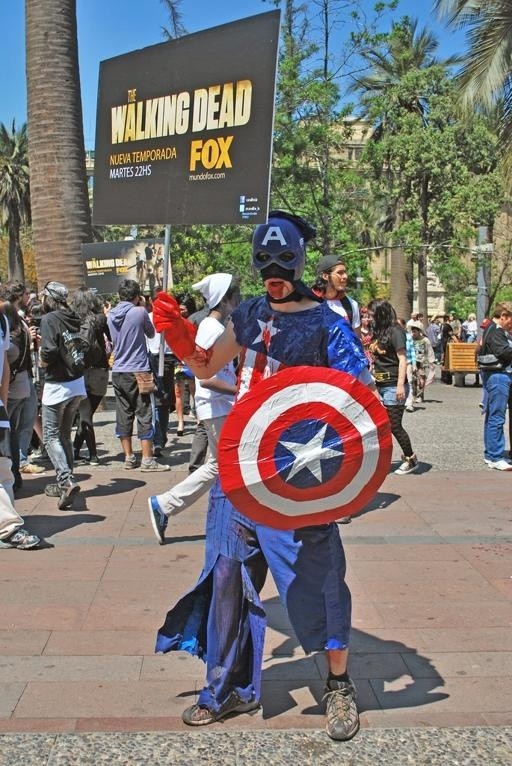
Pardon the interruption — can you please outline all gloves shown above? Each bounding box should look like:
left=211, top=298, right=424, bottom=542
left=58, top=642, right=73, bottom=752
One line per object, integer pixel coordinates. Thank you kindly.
left=150, top=288, right=202, bottom=364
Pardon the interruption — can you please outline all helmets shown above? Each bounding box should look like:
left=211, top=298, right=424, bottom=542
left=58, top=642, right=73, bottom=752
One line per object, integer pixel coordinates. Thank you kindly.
left=250, top=217, right=305, bottom=280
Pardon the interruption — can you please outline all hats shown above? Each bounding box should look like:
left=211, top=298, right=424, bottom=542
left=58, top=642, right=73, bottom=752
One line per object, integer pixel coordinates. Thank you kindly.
left=318, top=254, right=344, bottom=271
left=494, top=300, right=512, bottom=316
left=192, top=273, right=233, bottom=311
left=409, top=321, right=425, bottom=333
left=41, top=281, right=69, bottom=303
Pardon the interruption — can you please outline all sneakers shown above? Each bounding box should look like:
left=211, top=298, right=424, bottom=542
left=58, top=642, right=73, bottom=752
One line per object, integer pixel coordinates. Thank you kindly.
left=483, top=456, right=512, bottom=472
left=395, top=454, right=419, bottom=476
left=73, top=456, right=86, bottom=464
left=124, top=457, right=141, bottom=470
left=19, top=465, right=46, bottom=475
left=150, top=422, right=186, bottom=459
left=140, top=459, right=170, bottom=472
left=323, top=677, right=359, bottom=741
left=1, top=529, right=44, bottom=551
left=181, top=687, right=260, bottom=725
left=85, top=457, right=101, bottom=467
left=147, top=497, right=168, bottom=545
left=403, top=396, right=425, bottom=413
left=59, top=480, right=80, bottom=511
left=44, top=483, right=61, bottom=497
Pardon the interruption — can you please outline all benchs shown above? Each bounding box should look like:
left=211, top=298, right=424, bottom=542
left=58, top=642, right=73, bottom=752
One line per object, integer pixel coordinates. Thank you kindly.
left=449, top=342, right=485, bottom=386
left=440, top=342, right=451, bottom=382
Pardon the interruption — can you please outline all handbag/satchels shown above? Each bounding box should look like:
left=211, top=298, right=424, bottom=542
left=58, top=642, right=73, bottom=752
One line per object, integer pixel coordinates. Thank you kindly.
left=135, top=368, right=159, bottom=397
left=60, top=330, right=96, bottom=378
left=476, top=353, right=504, bottom=371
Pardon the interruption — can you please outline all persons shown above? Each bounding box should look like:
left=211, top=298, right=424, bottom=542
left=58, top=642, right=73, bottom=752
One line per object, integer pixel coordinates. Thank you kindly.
left=153, top=212, right=377, bottom=742
left=0, top=255, right=510, bottom=551
